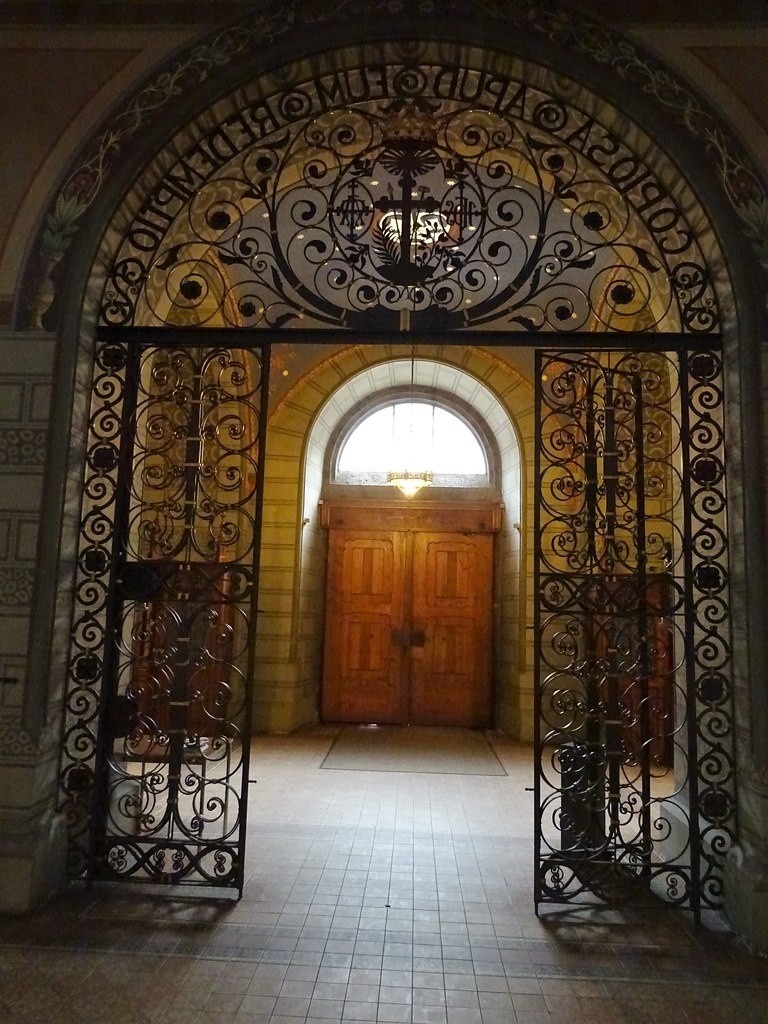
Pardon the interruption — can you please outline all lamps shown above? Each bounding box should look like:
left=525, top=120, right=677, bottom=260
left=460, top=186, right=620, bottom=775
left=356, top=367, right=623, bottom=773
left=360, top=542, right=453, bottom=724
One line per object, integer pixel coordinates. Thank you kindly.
left=387, top=402, right=434, bottom=501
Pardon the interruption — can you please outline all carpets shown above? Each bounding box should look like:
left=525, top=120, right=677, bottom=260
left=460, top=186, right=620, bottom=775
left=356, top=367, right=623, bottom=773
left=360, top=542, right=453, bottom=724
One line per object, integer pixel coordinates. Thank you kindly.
left=319, top=724, right=508, bottom=776
